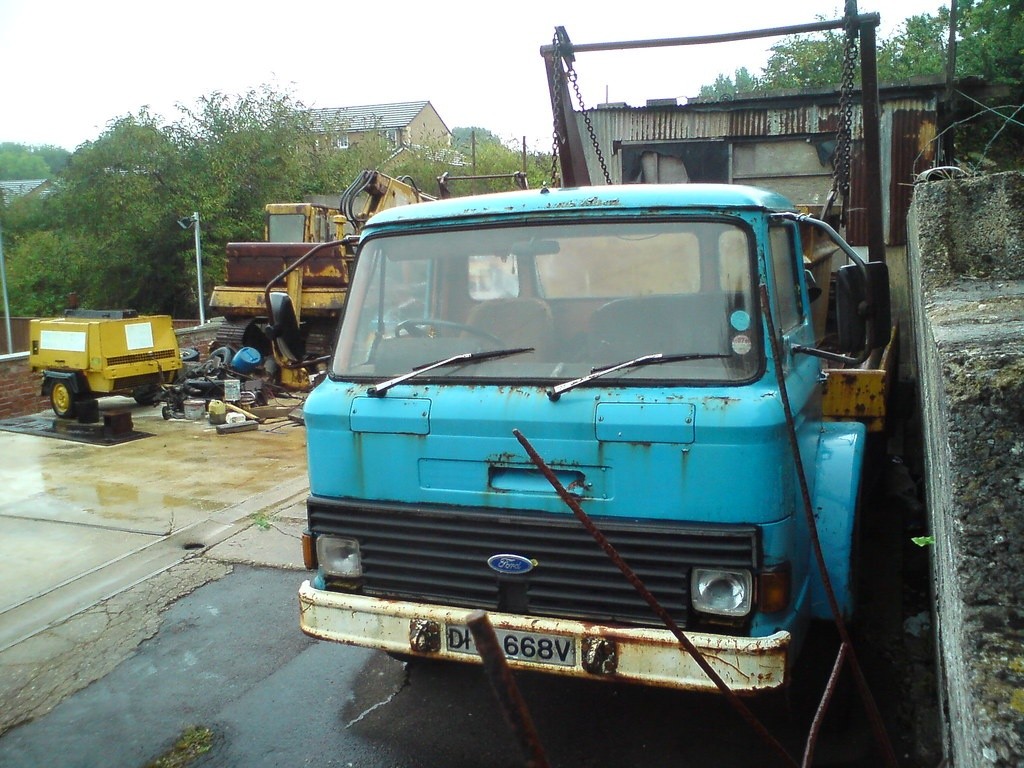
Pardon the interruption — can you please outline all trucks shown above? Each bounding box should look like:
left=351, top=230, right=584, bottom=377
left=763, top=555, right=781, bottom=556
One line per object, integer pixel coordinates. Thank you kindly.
left=292, top=181, right=902, bottom=708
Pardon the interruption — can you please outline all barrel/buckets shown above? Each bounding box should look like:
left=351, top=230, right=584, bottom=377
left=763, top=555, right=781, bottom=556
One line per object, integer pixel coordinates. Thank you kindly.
left=183, top=398, right=207, bottom=420
left=208, top=399, right=227, bottom=424
left=231, top=347, right=262, bottom=373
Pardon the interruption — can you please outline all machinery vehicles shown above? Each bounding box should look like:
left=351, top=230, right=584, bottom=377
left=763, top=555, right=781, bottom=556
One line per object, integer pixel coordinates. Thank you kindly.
left=208, top=170, right=443, bottom=391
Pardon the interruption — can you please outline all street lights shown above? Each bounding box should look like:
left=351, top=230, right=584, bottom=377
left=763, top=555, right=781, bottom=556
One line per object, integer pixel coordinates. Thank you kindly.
left=178, top=217, right=205, bottom=327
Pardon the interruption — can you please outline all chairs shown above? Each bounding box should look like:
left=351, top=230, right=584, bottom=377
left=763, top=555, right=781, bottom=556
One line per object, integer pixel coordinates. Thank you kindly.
left=593, top=296, right=672, bottom=356
left=455, top=295, right=557, bottom=375
